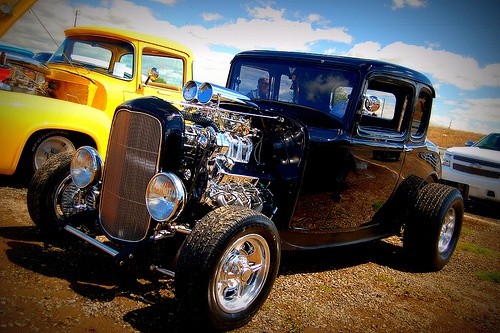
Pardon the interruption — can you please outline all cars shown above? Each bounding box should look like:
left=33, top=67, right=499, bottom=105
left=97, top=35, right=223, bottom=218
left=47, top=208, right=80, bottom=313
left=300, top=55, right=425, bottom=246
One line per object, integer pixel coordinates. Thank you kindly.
left=440, top=133, right=500, bottom=206
left=26, top=49, right=466, bottom=328
left=0, top=0, right=197, bottom=176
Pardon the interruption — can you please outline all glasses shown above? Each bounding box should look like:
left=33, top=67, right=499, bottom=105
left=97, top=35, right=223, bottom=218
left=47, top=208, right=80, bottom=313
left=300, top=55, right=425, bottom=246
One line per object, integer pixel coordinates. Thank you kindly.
left=263, top=83, right=269, bottom=87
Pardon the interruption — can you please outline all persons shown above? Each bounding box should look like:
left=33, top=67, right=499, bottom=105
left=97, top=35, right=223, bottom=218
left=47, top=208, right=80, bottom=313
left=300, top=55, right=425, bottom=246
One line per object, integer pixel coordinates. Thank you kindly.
left=246, top=76, right=269, bottom=98
